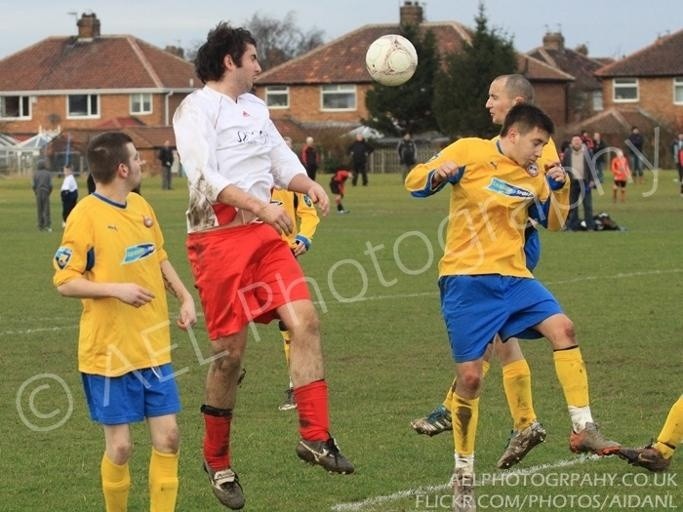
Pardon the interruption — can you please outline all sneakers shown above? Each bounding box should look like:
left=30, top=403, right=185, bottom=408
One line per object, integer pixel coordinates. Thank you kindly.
left=203, top=454, right=246, bottom=511
left=570, top=422, right=624, bottom=455
left=496, top=421, right=549, bottom=470
left=614, top=436, right=673, bottom=471
left=296, top=433, right=355, bottom=476
left=278, top=387, right=305, bottom=411
left=411, top=406, right=456, bottom=439
left=451, top=464, right=477, bottom=512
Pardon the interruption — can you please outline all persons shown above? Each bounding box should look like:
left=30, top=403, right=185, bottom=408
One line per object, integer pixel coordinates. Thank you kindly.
left=31, top=17, right=683, bottom=512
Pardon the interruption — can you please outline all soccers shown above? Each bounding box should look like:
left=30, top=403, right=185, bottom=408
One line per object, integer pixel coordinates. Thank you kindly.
left=364, top=33, right=420, bottom=87
